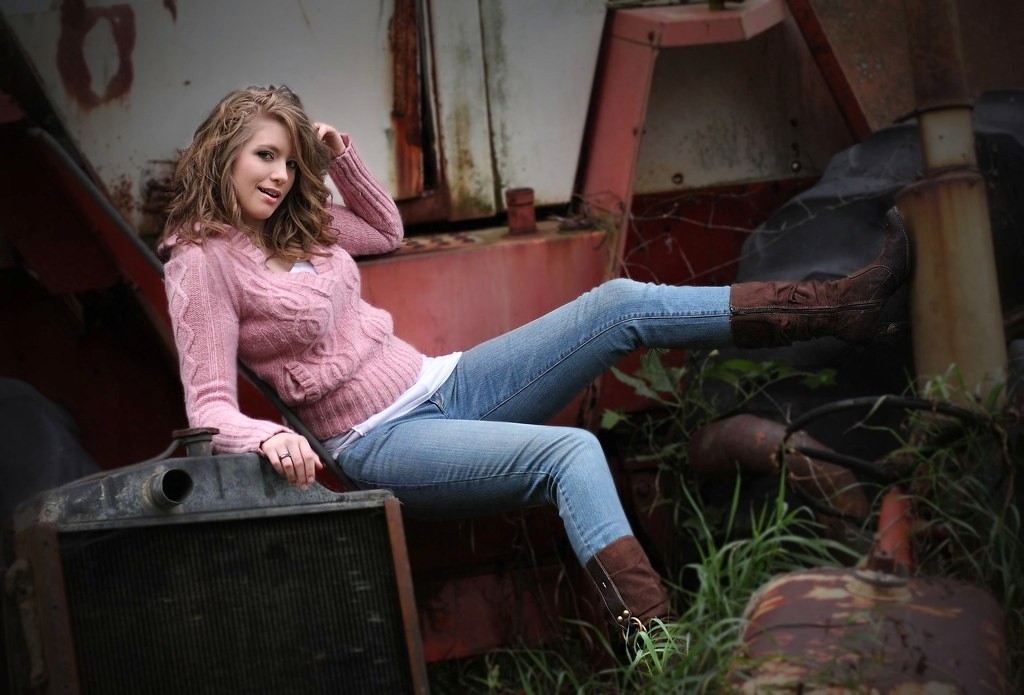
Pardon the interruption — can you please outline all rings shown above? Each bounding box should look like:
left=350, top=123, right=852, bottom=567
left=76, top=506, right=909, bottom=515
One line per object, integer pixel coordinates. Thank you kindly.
left=280, top=454, right=291, bottom=461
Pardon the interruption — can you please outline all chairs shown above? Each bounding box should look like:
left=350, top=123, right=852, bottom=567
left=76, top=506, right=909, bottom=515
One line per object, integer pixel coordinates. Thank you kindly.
left=0, top=125, right=579, bottom=576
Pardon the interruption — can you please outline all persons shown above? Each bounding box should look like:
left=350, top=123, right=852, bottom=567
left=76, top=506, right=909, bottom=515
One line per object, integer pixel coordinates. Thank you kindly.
left=158, top=83, right=910, bottom=673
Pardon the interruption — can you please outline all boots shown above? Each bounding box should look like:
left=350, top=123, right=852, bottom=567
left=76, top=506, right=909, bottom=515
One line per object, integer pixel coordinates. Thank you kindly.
left=586, top=536, right=691, bottom=686
left=728, top=210, right=913, bottom=350
